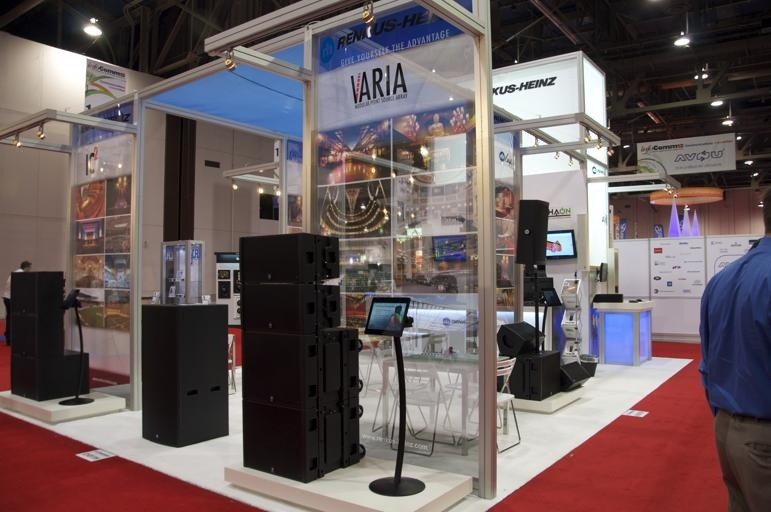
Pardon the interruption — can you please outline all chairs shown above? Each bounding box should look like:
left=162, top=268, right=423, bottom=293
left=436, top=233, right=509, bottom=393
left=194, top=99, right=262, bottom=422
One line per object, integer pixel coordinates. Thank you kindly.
left=359, top=328, right=521, bottom=456
left=228, top=334, right=237, bottom=394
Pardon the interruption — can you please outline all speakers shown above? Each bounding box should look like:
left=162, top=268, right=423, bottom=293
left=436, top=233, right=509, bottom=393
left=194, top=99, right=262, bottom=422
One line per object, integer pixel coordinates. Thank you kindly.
left=517, top=200, right=550, bottom=265
left=560, top=361, right=592, bottom=392
left=497, top=351, right=560, bottom=401
left=497, top=321, right=546, bottom=359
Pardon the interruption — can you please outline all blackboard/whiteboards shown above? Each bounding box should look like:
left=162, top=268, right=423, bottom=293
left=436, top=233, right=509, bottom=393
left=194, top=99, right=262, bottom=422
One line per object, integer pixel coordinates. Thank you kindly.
left=614, top=234, right=766, bottom=301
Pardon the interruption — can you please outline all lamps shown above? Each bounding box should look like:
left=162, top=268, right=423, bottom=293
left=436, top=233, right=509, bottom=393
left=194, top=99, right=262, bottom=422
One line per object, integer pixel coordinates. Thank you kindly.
left=37, top=125, right=46, bottom=140
left=12, top=131, right=24, bottom=149
left=81, top=18, right=104, bottom=37
left=223, top=49, right=237, bottom=71
left=651, top=12, right=766, bottom=212
left=534, top=137, right=575, bottom=167
left=360, top=1, right=376, bottom=26
left=583, top=130, right=630, bottom=156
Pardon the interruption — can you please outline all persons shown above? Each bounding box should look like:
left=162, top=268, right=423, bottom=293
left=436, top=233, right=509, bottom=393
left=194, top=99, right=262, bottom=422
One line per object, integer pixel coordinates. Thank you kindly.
left=496, top=264, right=514, bottom=287
left=389, top=305, right=401, bottom=328
left=2, top=261, right=32, bottom=346
left=429, top=261, right=457, bottom=293
left=698, top=189, right=771, bottom=512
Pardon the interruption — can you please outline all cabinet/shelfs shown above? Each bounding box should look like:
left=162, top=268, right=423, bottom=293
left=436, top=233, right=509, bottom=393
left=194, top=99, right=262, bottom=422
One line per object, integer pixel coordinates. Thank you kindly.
left=311, top=0, right=496, bottom=295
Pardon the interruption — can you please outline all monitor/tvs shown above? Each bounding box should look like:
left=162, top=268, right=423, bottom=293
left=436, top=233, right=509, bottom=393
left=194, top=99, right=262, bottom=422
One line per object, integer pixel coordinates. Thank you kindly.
left=62, top=290, right=80, bottom=310
left=364, top=297, right=410, bottom=337
left=600, top=263, right=608, bottom=282
left=524, top=277, right=553, bottom=301
left=542, top=287, right=562, bottom=306
left=545, top=230, right=577, bottom=259
left=431, top=235, right=467, bottom=262
